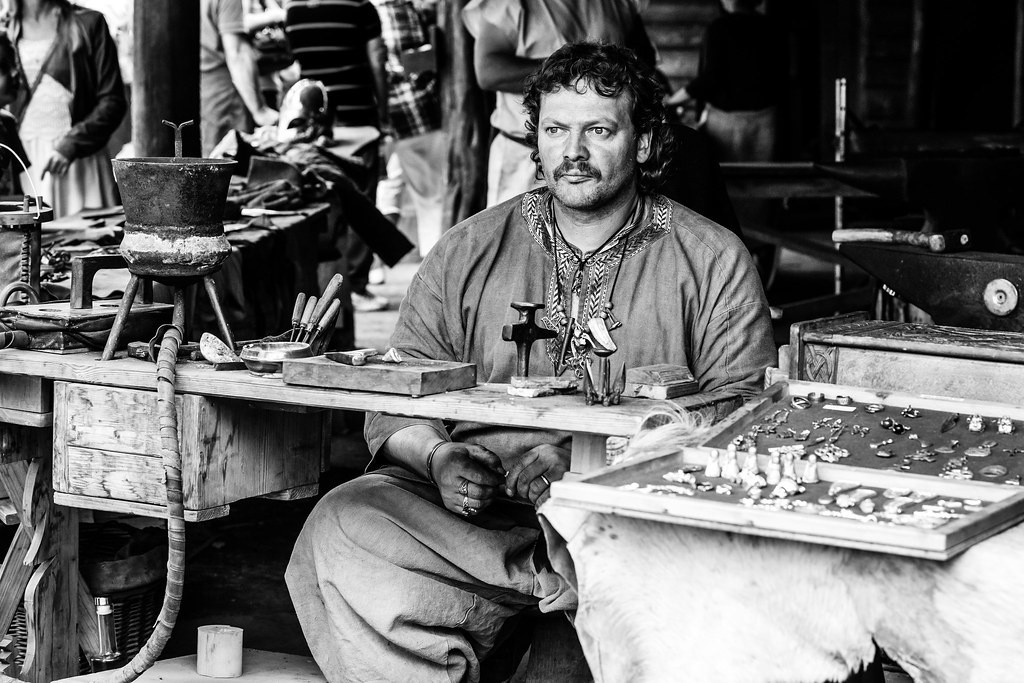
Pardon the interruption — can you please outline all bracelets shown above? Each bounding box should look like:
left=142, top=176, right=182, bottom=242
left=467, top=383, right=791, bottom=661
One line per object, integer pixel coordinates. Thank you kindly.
left=427, top=439, right=449, bottom=488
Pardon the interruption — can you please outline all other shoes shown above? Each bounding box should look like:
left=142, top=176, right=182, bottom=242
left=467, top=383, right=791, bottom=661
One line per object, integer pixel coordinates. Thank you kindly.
left=368, top=266, right=386, bottom=285
left=350, top=289, right=388, bottom=312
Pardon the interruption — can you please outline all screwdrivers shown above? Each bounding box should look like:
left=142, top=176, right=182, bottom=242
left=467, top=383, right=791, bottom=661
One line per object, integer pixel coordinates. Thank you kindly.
left=290, top=273, right=344, bottom=344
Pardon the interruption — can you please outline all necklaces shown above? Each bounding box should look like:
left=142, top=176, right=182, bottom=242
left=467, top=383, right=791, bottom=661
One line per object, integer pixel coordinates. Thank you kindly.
left=542, top=183, right=644, bottom=379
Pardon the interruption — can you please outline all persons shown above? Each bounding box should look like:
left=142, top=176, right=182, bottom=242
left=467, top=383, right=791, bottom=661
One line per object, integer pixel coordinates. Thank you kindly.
left=461, top=0, right=660, bottom=211
left=659, top=1, right=786, bottom=296
left=0, top=0, right=129, bottom=230
left=282, top=41, right=779, bottom=683
left=199, top=0, right=461, bottom=312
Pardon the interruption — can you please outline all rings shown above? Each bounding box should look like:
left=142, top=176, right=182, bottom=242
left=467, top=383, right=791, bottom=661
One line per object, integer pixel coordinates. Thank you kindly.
left=790, top=395, right=812, bottom=410
left=458, top=479, right=470, bottom=496
left=808, top=392, right=825, bottom=403
left=462, top=495, right=469, bottom=510
left=836, top=395, right=852, bottom=406
left=864, top=403, right=885, bottom=414
left=461, top=507, right=477, bottom=519
left=539, top=474, right=551, bottom=486
